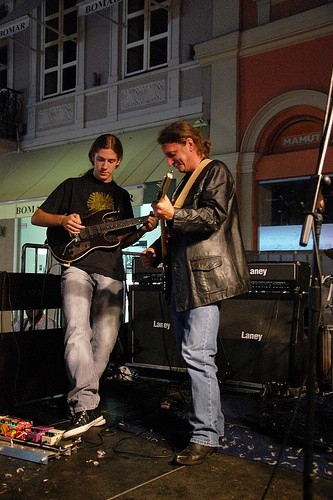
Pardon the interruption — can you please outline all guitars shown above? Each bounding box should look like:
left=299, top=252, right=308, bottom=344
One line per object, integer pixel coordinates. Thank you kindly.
left=46, top=208, right=161, bottom=264
left=153, top=170, right=174, bottom=256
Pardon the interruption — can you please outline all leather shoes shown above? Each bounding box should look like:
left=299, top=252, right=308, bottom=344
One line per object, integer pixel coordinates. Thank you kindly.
left=174, top=442, right=218, bottom=465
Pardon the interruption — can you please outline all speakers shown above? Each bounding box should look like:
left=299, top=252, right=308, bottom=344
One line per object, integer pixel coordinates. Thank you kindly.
left=128, top=280, right=318, bottom=398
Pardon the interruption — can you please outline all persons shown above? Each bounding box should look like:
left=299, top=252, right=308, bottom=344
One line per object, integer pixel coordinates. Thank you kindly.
left=31, top=134, right=159, bottom=438
left=140, top=122, right=254, bottom=463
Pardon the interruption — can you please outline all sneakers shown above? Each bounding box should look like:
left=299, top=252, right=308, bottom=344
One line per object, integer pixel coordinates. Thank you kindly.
left=63, top=408, right=106, bottom=441
